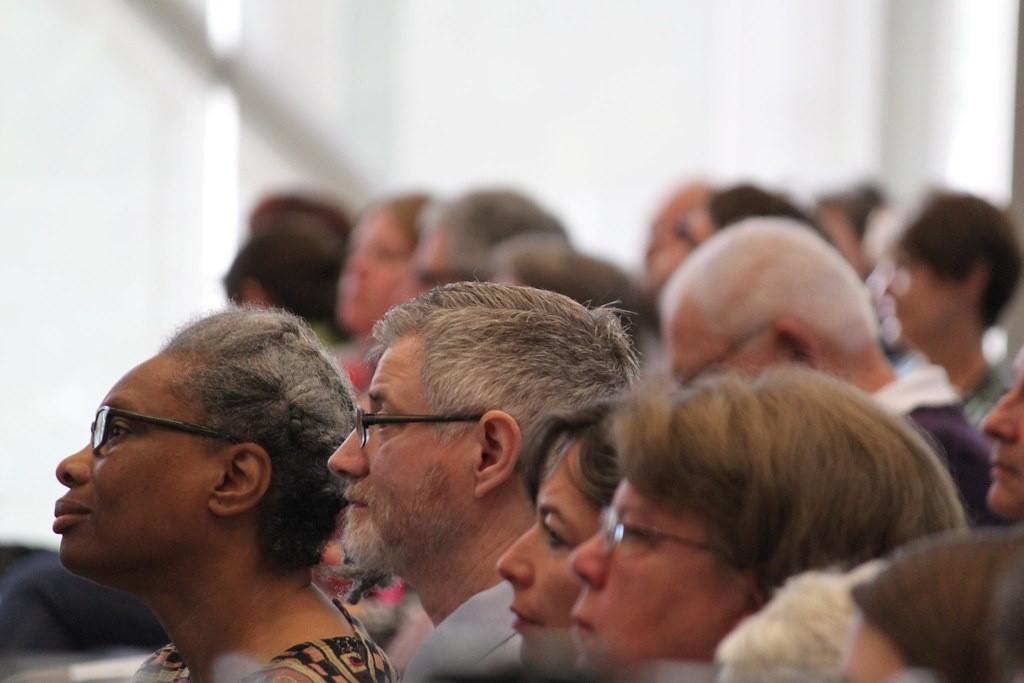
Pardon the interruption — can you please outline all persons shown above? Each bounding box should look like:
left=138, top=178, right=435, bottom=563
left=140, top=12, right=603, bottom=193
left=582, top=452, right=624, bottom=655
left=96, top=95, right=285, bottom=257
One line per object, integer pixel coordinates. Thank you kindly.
left=1, top=176, right=1024, bottom=683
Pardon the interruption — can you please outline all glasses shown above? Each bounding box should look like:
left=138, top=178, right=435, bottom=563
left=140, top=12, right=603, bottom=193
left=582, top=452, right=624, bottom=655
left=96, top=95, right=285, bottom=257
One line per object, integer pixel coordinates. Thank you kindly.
left=595, top=504, right=746, bottom=571
left=351, top=404, right=485, bottom=449
left=666, top=325, right=772, bottom=390
left=89, top=405, right=241, bottom=455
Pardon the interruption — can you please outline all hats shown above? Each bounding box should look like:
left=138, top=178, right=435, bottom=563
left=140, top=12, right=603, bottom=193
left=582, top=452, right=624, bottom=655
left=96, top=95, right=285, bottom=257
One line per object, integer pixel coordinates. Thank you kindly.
left=223, top=194, right=354, bottom=320
